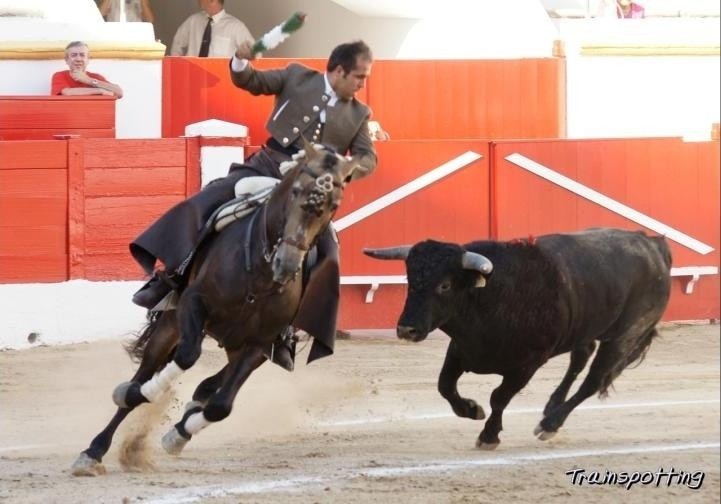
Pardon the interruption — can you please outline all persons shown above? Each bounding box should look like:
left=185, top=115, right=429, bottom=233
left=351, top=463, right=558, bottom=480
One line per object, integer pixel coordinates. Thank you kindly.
left=99, top=0, right=154, bottom=21
left=170, top=0, right=264, bottom=59
left=52, top=40, right=123, bottom=99
left=131, top=37, right=379, bottom=373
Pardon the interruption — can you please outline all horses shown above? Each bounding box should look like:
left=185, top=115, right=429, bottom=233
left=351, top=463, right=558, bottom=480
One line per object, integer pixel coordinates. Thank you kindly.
left=69, top=131, right=368, bottom=480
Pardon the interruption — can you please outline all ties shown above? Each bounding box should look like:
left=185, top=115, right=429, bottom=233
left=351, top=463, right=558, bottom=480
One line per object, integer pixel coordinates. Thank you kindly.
left=199, top=17, right=213, bottom=57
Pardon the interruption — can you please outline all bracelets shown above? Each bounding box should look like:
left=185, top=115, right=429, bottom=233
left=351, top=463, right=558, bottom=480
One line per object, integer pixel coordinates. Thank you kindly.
left=91, top=78, right=101, bottom=87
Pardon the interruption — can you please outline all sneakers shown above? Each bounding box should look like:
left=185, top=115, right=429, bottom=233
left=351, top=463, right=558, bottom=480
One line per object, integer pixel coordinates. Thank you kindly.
left=264, top=335, right=294, bottom=371
left=132, top=277, right=170, bottom=308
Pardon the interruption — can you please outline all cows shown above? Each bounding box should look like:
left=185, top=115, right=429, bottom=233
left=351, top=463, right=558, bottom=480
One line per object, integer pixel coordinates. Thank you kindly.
left=361, top=224, right=675, bottom=452
